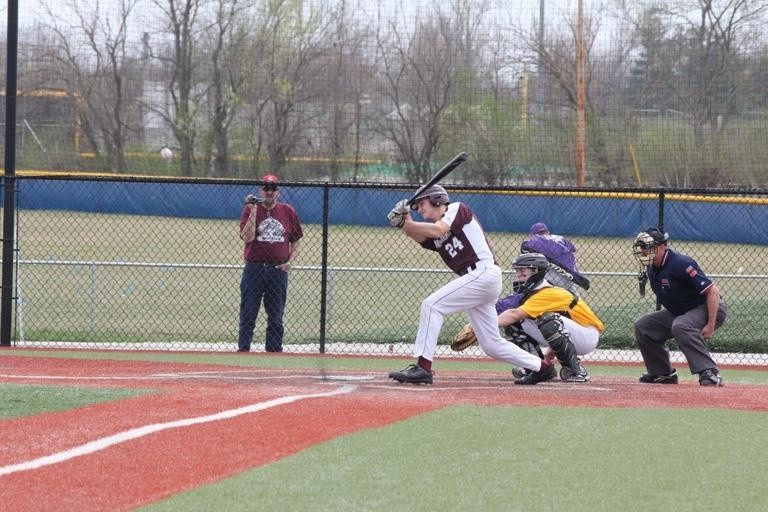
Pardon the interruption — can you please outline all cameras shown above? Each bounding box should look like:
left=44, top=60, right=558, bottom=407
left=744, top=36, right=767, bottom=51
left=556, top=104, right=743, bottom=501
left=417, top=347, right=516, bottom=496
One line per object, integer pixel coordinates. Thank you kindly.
left=249, top=196, right=264, bottom=203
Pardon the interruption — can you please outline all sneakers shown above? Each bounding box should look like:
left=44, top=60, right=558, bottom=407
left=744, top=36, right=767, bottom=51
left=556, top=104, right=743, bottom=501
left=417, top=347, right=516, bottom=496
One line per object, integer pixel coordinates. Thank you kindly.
left=512, top=363, right=589, bottom=384
left=639, top=368, right=678, bottom=384
left=389, top=364, right=433, bottom=384
left=699, top=369, right=722, bottom=385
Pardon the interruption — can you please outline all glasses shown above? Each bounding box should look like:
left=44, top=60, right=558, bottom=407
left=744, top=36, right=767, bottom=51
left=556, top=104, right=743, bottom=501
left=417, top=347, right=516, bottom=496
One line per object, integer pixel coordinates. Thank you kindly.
left=263, top=185, right=277, bottom=191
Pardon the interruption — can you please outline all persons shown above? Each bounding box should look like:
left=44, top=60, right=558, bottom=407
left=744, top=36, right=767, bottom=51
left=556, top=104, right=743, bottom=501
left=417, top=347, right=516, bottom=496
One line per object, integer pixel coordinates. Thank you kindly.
left=512, top=223, right=578, bottom=378
left=387, top=184, right=557, bottom=384
left=237, top=175, right=303, bottom=353
left=632, top=228, right=728, bottom=387
left=450, top=253, right=604, bottom=382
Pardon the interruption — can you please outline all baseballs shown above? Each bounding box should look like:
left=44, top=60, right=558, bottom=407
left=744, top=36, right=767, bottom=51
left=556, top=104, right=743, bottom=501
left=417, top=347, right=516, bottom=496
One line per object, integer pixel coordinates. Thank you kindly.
left=161, top=147, right=172, bottom=159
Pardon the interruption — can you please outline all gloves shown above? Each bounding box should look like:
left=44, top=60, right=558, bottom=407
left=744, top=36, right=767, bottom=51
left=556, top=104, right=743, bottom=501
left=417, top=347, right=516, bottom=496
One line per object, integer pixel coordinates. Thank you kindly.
left=395, top=199, right=410, bottom=214
left=387, top=209, right=403, bottom=226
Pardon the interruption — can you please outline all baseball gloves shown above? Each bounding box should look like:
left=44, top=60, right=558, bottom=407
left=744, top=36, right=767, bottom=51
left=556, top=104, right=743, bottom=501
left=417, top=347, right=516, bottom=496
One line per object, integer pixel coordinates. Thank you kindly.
left=451, top=323, right=476, bottom=351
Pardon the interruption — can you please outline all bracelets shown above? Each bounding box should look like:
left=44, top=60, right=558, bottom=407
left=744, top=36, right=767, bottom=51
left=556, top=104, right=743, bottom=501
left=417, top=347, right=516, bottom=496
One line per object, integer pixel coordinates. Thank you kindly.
left=287, top=261, right=291, bottom=266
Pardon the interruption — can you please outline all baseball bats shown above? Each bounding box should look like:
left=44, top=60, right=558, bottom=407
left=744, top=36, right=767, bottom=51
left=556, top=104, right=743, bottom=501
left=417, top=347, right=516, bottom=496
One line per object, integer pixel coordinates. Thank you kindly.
left=407, top=151, right=470, bottom=207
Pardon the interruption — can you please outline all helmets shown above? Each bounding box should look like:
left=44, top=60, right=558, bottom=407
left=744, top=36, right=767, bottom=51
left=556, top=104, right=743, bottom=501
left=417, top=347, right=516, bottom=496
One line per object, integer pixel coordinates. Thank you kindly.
left=415, top=185, right=449, bottom=206
left=631, top=227, right=667, bottom=273
left=512, top=252, right=548, bottom=294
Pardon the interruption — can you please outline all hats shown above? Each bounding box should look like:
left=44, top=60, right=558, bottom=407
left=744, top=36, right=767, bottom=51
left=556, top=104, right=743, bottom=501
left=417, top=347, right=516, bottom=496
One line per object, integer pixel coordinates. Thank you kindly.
left=263, top=175, right=278, bottom=182
left=530, top=223, right=547, bottom=234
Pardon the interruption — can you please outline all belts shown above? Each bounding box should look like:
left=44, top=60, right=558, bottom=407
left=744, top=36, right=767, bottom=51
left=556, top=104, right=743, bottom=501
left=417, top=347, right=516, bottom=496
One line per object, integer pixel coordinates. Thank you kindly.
left=251, top=261, right=284, bottom=267
left=459, top=263, right=476, bottom=276
